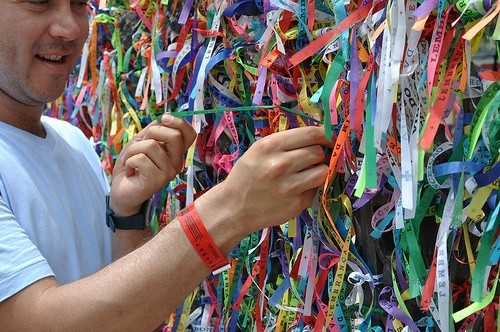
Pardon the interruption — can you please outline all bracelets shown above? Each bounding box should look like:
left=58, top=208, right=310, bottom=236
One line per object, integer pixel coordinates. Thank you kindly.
left=176, top=202, right=232, bottom=276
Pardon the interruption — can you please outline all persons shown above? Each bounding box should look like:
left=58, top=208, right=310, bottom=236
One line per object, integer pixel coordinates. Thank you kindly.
left=1, top=1, right=332, bottom=331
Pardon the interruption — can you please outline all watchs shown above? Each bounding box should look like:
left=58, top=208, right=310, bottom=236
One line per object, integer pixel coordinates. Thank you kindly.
left=105, top=189, right=157, bottom=232
